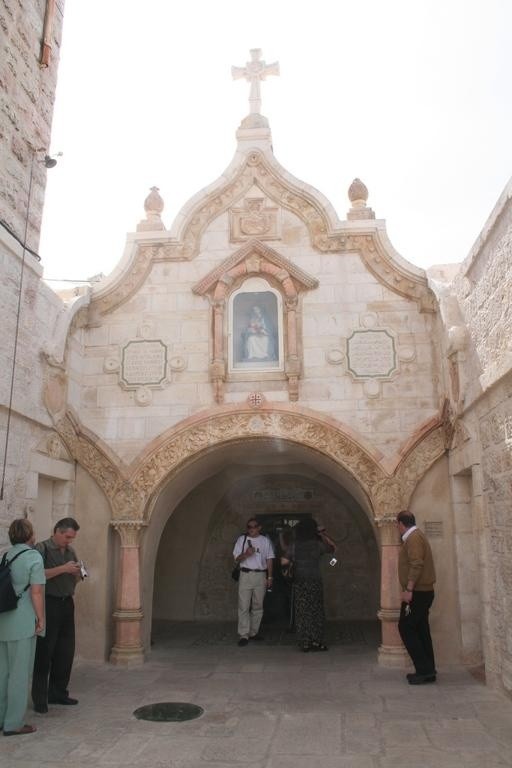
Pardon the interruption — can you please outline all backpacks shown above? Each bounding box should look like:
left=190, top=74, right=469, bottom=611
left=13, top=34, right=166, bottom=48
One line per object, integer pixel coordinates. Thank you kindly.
left=0, top=548, right=36, bottom=613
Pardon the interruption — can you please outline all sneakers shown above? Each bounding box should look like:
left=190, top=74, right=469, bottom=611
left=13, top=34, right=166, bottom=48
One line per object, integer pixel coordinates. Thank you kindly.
left=35, top=702, right=49, bottom=713
left=247, top=634, right=265, bottom=641
left=238, top=638, right=248, bottom=646
left=407, top=672, right=436, bottom=684
left=48, top=697, right=78, bottom=705
left=0, top=724, right=37, bottom=735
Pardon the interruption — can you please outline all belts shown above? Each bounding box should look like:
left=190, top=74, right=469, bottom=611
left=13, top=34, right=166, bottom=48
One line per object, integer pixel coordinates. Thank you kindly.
left=241, top=567, right=267, bottom=572
left=45, top=594, right=73, bottom=601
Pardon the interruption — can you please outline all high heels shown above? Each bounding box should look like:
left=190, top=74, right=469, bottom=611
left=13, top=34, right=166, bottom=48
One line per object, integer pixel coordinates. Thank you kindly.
left=303, top=640, right=328, bottom=652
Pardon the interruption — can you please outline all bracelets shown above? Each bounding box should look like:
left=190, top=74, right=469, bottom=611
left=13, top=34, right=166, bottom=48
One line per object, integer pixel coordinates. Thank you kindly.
left=406, top=588, right=413, bottom=592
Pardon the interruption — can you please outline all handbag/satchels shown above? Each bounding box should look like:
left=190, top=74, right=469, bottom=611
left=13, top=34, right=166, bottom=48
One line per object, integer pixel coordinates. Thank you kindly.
left=278, top=560, right=294, bottom=581
left=232, top=565, right=239, bottom=580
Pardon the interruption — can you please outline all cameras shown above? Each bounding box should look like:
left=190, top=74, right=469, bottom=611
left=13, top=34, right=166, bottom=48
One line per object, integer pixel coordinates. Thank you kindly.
left=74, top=560, right=88, bottom=582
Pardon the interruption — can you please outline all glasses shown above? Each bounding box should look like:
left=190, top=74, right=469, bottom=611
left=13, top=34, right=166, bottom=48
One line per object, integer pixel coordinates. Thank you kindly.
left=247, top=524, right=259, bottom=529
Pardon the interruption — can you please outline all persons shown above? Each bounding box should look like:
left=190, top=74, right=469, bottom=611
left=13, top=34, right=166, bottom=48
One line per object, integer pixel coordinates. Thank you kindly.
left=30, top=518, right=84, bottom=713
left=231, top=518, right=337, bottom=653
left=0, top=519, right=47, bottom=736
left=396, top=510, right=437, bottom=685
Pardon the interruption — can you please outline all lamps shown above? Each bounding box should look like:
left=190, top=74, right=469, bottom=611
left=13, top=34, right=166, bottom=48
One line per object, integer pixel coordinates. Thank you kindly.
left=36, top=147, right=57, bottom=168
